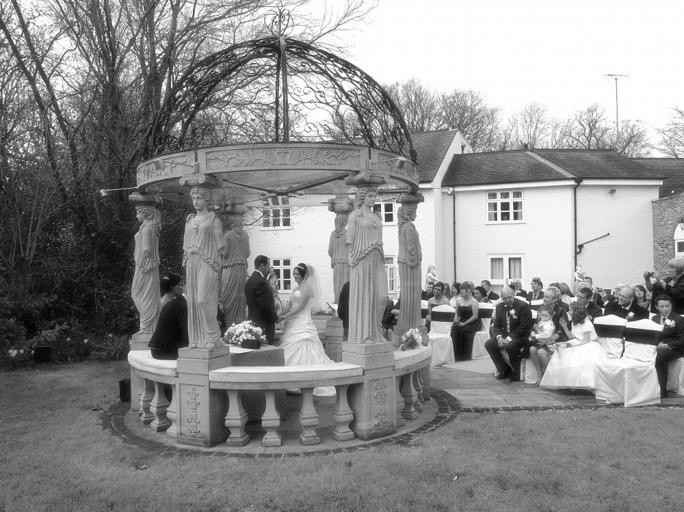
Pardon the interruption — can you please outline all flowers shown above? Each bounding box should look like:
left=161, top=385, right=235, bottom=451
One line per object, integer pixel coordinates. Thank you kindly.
left=223, top=318, right=268, bottom=346
left=398, top=326, right=423, bottom=350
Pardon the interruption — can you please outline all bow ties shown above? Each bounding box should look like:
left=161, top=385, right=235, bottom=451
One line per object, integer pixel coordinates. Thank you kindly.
left=505, top=306, right=513, bottom=311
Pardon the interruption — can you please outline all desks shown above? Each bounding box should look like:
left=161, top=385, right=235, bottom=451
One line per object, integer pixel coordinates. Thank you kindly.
left=220, top=336, right=284, bottom=366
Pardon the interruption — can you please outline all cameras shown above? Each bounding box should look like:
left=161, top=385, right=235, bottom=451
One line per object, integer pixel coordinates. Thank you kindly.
left=647, top=271, right=655, bottom=277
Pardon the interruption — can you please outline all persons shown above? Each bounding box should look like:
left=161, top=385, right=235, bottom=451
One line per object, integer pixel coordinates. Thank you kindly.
left=426, top=267, right=439, bottom=284
left=148, top=273, right=187, bottom=360
left=182, top=187, right=252, bottom=349
left=244, top=255, right=278, bottom=346
left=574, top=265, right=589, bottom=284
left=130, top=205, right=161, bottom=336
left=642, top=257, right=683, bottom=314
left=327, top=186, right=390, bottom=343
left=397, top=203, right=422, bottom=331
left=278, top=263, right=339, bottom=396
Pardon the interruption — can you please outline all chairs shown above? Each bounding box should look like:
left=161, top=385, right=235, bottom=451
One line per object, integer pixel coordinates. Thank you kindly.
left=420, top=295, right=684, bottom=411
